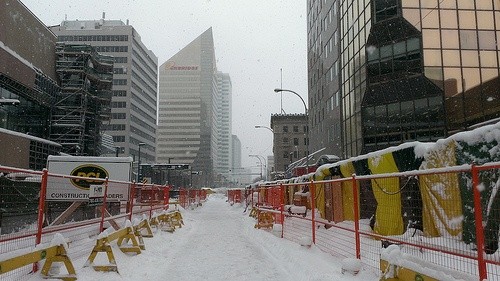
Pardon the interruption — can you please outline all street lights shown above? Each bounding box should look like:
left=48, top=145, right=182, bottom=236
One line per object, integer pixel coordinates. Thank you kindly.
left=274, top=89, right=308, bottom=173
left=250, top=154, right=263, bottom=179
left=253, top=155, right=267, bottom=182
left=254, top=125, right=277, bottom=180
left=168, top=157, right=173, bottom=165
left=138, top=142, right=146, bottom=184
